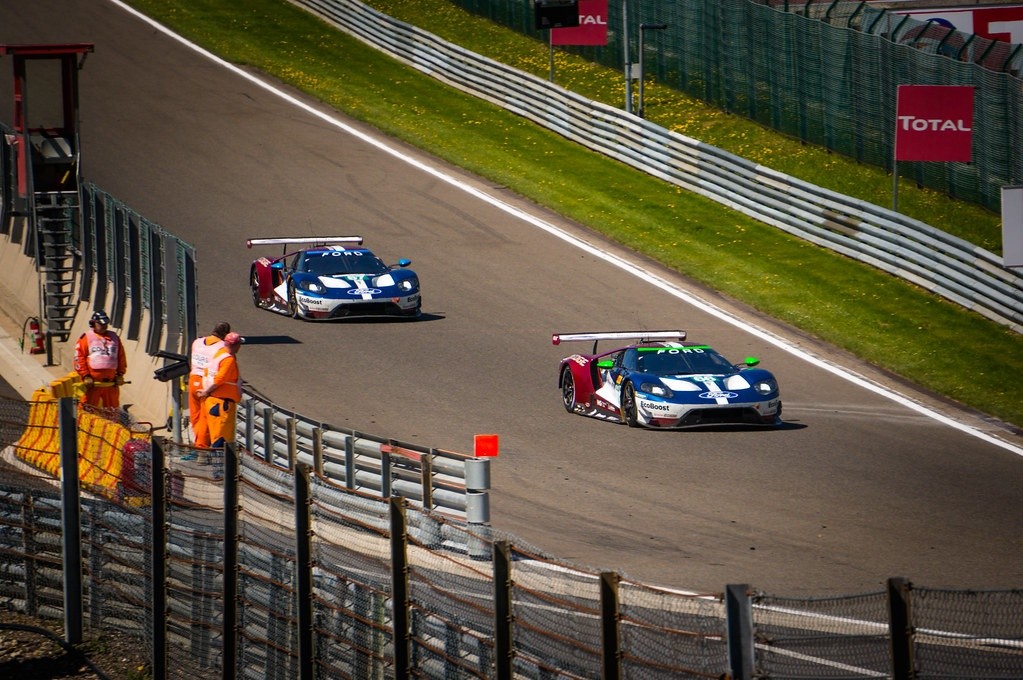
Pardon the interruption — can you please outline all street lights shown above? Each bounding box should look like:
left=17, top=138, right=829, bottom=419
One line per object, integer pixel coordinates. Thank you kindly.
left=636, top=23, right=669, bottom=118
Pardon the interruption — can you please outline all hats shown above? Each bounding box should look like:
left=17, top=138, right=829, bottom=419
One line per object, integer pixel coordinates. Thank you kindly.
left=92, top=311, right=109, bottom=325
left=224, top=332, right=245, bottom=345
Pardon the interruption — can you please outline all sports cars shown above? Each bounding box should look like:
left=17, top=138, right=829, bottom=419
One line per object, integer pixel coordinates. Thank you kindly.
left=551, top=329, right=785, bottom=432
left=245, top=234, right=424, bottom=324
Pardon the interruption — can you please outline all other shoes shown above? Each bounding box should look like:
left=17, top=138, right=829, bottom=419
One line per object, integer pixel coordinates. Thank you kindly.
left=197, top=452, right=212, bottom=467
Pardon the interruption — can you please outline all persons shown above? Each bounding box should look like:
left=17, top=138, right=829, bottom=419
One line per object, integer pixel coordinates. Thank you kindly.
left=188, top=321, right=231, bottom=466
left=74, top=311, right=128, bottom=421
left=196, top=333, right=245, bottom=482
